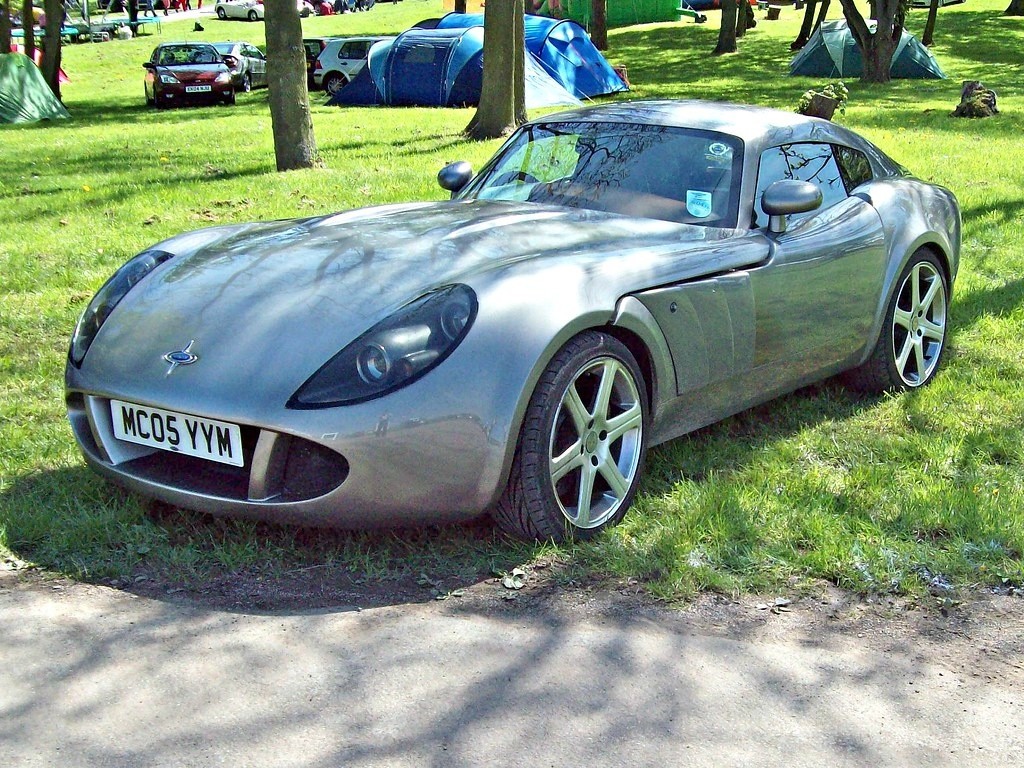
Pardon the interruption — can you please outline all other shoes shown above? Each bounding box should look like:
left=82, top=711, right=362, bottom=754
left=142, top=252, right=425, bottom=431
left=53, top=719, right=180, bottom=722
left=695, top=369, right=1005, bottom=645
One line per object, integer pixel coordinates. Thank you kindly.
left=166, top=14, right=168, bottom=16
left=144, top=14, right=148, bottom=17
left=163, top=11, right=165, bottom=15
left=154, top=15, right=157, bottom=17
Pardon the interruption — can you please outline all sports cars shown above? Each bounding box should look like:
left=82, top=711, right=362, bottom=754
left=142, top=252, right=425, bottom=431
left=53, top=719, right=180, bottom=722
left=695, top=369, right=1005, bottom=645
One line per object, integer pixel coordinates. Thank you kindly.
left=63, top=96, right=964, bottom=547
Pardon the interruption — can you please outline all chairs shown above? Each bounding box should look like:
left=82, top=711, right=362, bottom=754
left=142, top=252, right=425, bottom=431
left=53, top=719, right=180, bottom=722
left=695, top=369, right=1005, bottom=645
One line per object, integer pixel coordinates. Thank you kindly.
left=10, top=15, right=161, bottom=48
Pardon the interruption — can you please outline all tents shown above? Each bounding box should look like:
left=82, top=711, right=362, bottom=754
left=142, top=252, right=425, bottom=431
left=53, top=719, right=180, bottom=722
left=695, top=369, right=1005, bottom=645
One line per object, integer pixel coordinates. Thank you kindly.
left=786, top=19, right=947, bottom=80
left=322, top=26, right=596, bottom=110
left=10, top=44, right=71, bottom=84
left=411, top=12, right=632, bottom=100
left=0, top=51, right=73, bottom=124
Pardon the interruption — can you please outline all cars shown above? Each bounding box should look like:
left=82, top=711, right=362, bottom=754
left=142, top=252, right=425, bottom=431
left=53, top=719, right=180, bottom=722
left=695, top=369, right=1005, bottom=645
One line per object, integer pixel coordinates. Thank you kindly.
left=907, top=0, right=967, bottom=8
left=215, top=0, right=376, bottom=22
left=186, top=39, right=267, bottom=93
left=304, top=34, right=347, bottom=76
left=141, top=42, right=237, bottom=109
left=312, top=36, right=400, bottom=97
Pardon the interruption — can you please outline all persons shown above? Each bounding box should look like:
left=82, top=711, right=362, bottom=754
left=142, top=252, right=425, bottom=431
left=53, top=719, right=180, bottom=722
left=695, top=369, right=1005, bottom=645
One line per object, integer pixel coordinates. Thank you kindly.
left=144, top=0, right=157, bottom=17
left=162, top=0, right=170, bottom=16
left=175, top=0, right=202, bottom=13
